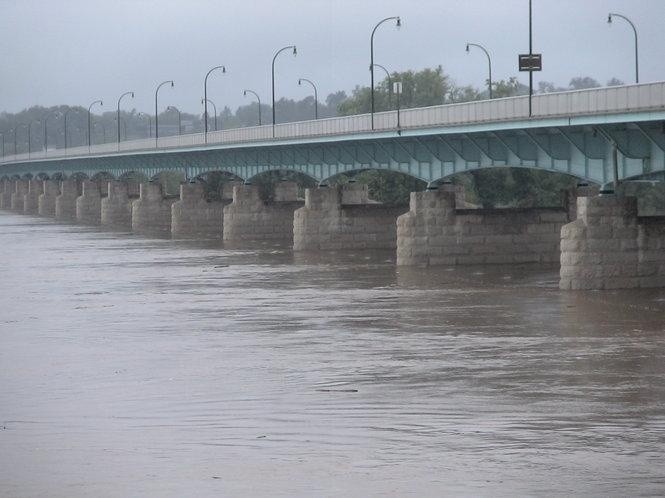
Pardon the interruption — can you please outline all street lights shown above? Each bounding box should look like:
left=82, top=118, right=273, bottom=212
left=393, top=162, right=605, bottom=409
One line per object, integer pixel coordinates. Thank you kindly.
left=272, top=45, right=297, bottom=124
left=298, top=78, right=318, bottom=119
left=243, top=89, right=261, bottom=127
left=369, top=16, right=402, bottom=112
left=466, top=42, right=494, bottom=99
left=201, top=97, right=217, bottom=130
left=204, top=65, right=225, bottom=132
left=369, top=63, right=392, bottom=110
left=607, top=13, right=640, bottom=84
left=0, top=80, right=182, bottom=158
